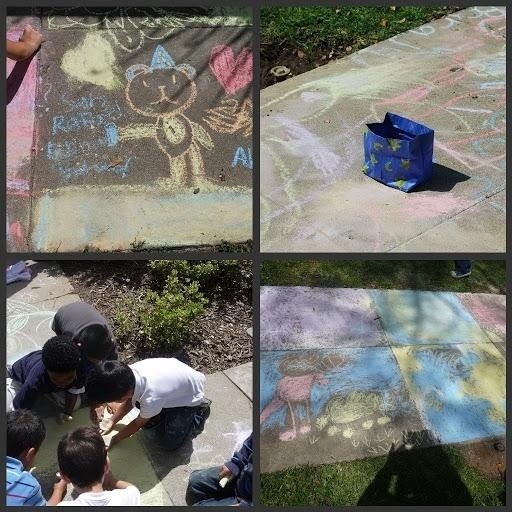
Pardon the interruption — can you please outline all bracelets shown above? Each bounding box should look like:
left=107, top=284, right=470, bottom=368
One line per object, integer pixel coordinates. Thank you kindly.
left=65, top=412, right=71, bottom=415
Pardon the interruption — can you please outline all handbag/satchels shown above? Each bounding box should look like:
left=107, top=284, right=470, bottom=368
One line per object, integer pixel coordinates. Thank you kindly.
left=361, top=111, right=435, bottom=194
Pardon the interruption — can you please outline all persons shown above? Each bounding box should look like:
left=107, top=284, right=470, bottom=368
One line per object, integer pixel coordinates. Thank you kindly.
left=7, top=24, right=43, bottom=61
left=52, top=301, right=121, bottom=423
left=6, top=334, right=87, bottom=422
left=88, top=357, right=213, bottom=451
left=6, top=411, right=67, bottom=506
left=54, top=425, right=141, bottom=506
left=188, top=432, right=254, bottom=507
